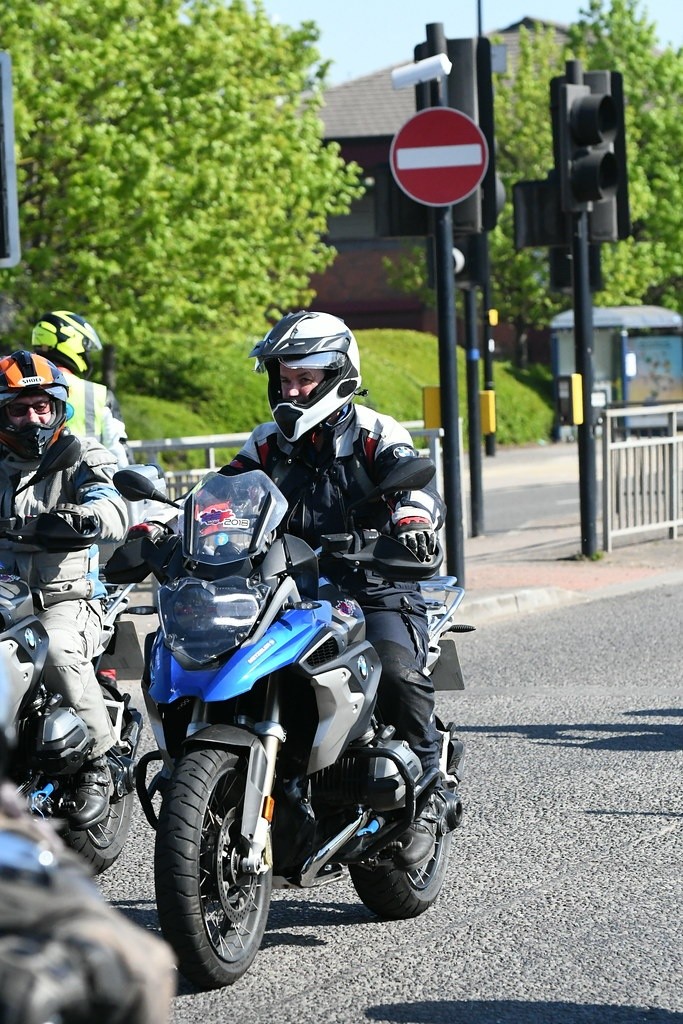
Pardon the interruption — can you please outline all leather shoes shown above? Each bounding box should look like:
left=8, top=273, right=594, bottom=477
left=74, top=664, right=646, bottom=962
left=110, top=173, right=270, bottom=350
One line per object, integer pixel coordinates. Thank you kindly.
left=64, top=758, right=117, bottom=832
left=387, top=789, right=449, bottom=870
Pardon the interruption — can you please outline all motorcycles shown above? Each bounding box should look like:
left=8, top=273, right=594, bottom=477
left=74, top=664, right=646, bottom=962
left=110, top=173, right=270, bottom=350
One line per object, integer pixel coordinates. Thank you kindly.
left=101, top=448, right=478, bottom=989
left=1, top=510, right=153, bottom=873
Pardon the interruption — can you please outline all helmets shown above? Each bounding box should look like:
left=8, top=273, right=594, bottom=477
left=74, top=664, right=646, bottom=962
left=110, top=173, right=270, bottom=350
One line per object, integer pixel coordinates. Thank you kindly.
left=247, top=310, right=362, bottom=443
left=31, top=309, right=102, bottom=381
left=0, top=351, right=68, bottom=460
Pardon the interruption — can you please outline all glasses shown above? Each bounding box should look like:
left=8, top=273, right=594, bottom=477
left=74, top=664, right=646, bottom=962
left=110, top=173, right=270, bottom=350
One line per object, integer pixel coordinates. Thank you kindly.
left=4, top=401, right=57, bottom=419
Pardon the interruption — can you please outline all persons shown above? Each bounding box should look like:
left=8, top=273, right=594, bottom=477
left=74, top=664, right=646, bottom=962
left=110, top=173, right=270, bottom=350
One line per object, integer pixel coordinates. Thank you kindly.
left=25, top=310, right=131, bottom=487
left=0, top=356, right=152, bottom=802
left=134, top=307, right=468, bottom=870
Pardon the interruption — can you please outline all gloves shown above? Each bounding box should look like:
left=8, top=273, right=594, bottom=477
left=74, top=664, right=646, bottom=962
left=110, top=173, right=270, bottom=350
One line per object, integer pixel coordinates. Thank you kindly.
left=378, top=496, right=439, bottom=564
left=119, top=516, right=176, bottom=553
left=49, top=500, right=95, bottom=537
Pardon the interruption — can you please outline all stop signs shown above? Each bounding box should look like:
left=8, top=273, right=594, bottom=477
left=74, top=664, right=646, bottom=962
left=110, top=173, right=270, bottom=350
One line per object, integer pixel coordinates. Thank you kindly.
left=389, top=106, right=492, bottom=209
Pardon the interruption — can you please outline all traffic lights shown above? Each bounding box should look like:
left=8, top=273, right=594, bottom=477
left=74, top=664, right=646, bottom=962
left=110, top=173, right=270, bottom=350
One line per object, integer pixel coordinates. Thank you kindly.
left=556, top=80, right=621, bottom=214
